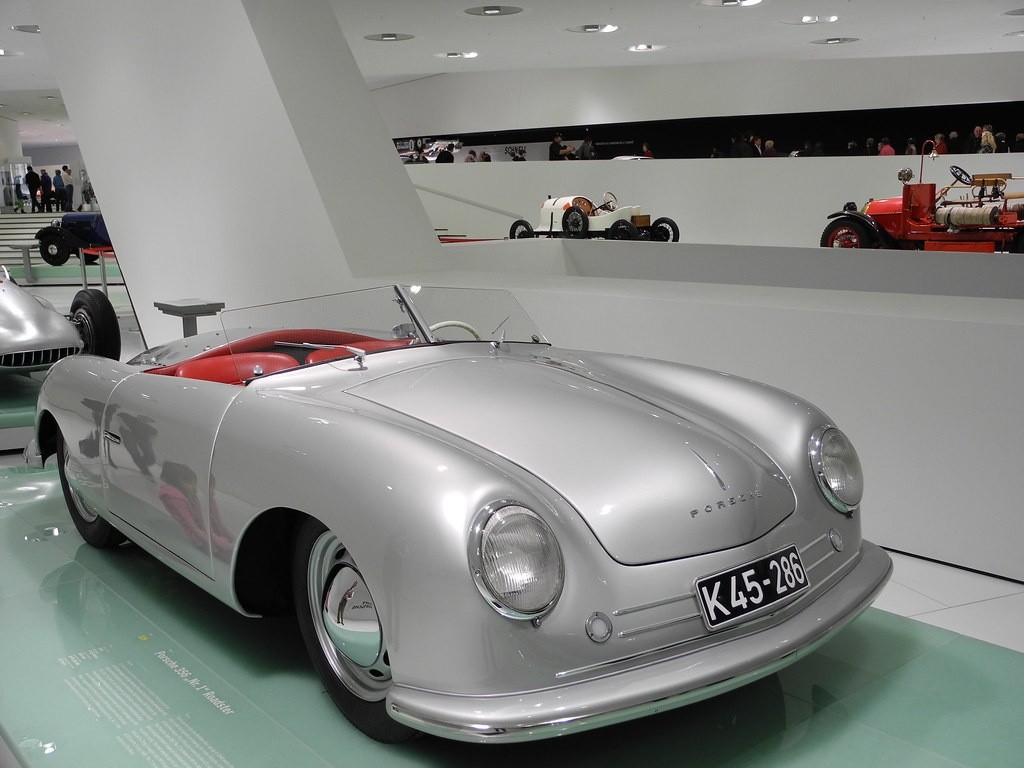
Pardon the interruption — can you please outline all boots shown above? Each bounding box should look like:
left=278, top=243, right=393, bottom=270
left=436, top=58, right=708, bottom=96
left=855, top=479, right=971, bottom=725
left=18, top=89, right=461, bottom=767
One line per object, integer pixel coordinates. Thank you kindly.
left=21, top=209, right=27, bottom=213
left=13, top=208, right=19, bottom=213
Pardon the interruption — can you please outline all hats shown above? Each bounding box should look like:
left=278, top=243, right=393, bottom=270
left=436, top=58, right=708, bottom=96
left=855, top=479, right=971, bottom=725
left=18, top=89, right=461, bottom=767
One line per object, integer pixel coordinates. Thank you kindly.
left=554, top=133, right=562, bottom=137
left=908, top=138, right=915, bottom=144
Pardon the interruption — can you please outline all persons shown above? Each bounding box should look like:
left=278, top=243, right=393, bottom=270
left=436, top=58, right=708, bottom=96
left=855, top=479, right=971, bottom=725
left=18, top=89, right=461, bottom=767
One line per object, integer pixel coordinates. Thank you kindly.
left=40, top=165, right=76, bottom=212
left=879, top=137, right=895, bottom=155
left=640, top=142, right=654, bottom=157
left=465, top=150, right=491, bottom=162
left=948, top=132, right=963, bottom=154
left=408, top=147, right=430, bottom=163
left=803, top=141, right=825, bottom=155
left=846, top=141, right=859, bottom=153
left=13, top=184, right=28, bottom=213
left=510, top=149, right=527, bottom=161
left=579, top=134, right=600, bottom=160
left=762, top=139, right=776, bottom=154
left=726, top=128, right=764, bottom=157
left=932, top=133, right=948, bottom=154
left=864, top=138, right=878, bottom=156
left=964, top=125, right=1024, bottom=154
left=25, top=167, right=41, bottom=212
left=435, top=144, right=455, bottom=163
left=548, top=132, right=576, bottom=160
left=905, top=138, right=917, bottom=155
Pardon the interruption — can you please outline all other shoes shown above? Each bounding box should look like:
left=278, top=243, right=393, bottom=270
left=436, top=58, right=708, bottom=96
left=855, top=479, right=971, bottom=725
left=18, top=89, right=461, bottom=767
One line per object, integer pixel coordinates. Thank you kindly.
left=56, top=209, right=59, bottom=212
left=31, top=211, right=35, bottom=213
left=70, top=210, right=76, bottom=212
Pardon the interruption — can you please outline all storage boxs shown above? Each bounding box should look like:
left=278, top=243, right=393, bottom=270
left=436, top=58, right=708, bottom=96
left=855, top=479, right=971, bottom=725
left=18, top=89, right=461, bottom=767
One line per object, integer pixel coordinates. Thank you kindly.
left=631, top=213, right=651, bottom=228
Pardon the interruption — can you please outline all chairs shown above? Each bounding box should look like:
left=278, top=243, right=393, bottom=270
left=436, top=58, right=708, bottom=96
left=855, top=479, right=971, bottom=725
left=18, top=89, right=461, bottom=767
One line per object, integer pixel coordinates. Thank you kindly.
left=306, top=340, right=411, bottom=364
left=176, top=352, right=299, bottom=385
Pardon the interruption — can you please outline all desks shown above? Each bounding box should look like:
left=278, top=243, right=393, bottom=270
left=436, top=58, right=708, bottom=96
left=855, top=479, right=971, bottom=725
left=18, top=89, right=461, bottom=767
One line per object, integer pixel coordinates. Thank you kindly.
left=8, top=242, right=39, bottom=282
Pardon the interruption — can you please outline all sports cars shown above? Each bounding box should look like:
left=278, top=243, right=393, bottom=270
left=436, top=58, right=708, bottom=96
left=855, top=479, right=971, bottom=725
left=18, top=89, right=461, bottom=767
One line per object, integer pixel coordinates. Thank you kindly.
left=24, top=285, right=894, bottom=745
left=0, top=263, right=121, bottom=373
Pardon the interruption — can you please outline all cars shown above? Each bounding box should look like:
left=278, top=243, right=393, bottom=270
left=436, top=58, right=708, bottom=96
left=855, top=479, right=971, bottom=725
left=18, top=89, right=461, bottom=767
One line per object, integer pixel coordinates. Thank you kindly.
left=610, top=155, right=654, bottom=160
left=789, top=150, right=807, bottom=157
left=34, top=212, right=116, bottom=266
left=821, top=139, right=1024, bottom=255
left=509, top=191, right=679, bottom=242
